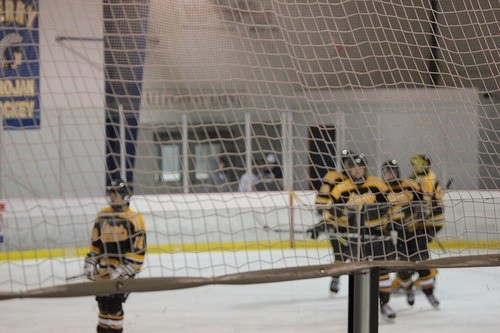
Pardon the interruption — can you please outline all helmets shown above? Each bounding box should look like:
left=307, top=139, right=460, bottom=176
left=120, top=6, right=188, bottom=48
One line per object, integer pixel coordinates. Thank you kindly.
left=107, top=170, right=134, bottom=210
left=341, top=150, right=367, bottom=185
left=412, top=152, right=431, bottom=176
left=381, top=160, right=400, bottom=183
left=411, top=155, right=432, bottom=166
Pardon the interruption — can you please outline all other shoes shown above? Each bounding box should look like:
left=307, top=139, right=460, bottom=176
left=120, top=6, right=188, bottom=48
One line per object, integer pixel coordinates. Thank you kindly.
left=408, top=293, right=414, bottom=305
left=381, top=304, right=397, bottom=323
left=330, top=278, right=339, bottom=293
left=427, top=294, right=440, bottom=311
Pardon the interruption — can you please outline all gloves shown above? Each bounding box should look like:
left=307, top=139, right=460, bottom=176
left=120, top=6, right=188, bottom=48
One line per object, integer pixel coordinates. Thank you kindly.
left=110, top=263, right=136, bottom=279
left=83, top=257, right=100, bottom=281
left=306, top=222, right=326, bottom=238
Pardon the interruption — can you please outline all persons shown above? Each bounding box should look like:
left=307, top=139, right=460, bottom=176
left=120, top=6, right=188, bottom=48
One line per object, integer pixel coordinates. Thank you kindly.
left=82, top=177, right=147, bottom=333
left=238, top=159, right=259, bottom=192
left=315, top=150, right=445, bottom=318
left=262, top=153, right=284, bottom=191
